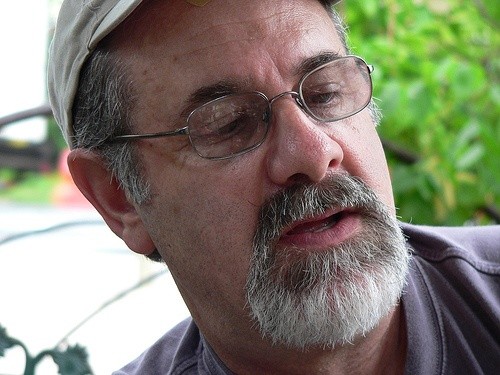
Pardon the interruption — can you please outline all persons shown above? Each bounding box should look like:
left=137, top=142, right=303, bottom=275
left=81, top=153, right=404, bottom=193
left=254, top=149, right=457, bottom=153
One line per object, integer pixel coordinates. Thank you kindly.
left=45, top=1, right=500, bottom=375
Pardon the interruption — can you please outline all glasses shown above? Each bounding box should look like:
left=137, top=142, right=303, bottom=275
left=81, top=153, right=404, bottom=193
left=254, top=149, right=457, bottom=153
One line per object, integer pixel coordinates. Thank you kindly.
left=102, top=52, right=376, bottom=161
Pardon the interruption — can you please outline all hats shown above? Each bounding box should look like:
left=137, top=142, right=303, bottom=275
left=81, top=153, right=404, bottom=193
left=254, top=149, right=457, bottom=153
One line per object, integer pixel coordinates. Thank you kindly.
left=44, top=1, right=345, bottom=151
